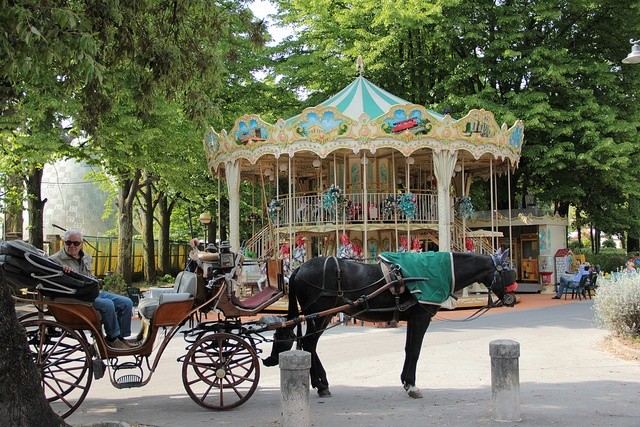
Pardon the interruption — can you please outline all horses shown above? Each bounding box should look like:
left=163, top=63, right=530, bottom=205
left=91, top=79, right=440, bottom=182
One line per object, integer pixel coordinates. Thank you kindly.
left=261, top=246, right=518, bottom=399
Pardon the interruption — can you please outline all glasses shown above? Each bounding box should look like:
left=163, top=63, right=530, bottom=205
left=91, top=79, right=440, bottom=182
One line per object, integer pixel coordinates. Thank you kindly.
left=63, top=240, right=81, bottom=246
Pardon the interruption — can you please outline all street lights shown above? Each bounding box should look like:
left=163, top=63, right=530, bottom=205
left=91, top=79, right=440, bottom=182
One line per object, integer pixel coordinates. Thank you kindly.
left=199, top=212, right=212, bottom=251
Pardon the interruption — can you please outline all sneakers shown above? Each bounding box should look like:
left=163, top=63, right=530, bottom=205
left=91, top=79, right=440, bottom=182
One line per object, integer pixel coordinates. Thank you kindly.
left=105, top=338, right=129, bottom=349
left=121, top=337, right=139, bottom=347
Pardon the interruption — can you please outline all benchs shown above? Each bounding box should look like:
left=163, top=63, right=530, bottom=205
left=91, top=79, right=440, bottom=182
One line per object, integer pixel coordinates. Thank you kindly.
left=48, top=259, right=284, bottom=329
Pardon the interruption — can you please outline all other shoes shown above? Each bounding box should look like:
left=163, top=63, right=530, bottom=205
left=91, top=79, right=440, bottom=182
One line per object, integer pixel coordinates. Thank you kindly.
left=552, top=296, right=560, bottom=299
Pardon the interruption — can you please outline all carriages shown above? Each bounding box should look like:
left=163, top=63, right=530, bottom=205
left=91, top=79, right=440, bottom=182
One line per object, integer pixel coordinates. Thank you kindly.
left=0, top=230, right=520, bottom=418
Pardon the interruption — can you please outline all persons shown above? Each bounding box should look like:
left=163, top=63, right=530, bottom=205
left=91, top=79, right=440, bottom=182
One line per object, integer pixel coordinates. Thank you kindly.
left=45, top=229, right=139, bottom=350
left=551, top=264, right=594, bottom=299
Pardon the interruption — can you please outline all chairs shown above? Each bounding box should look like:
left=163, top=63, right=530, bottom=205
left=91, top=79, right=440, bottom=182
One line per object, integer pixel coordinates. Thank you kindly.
left=564, top=273, right=600, bottom=300
